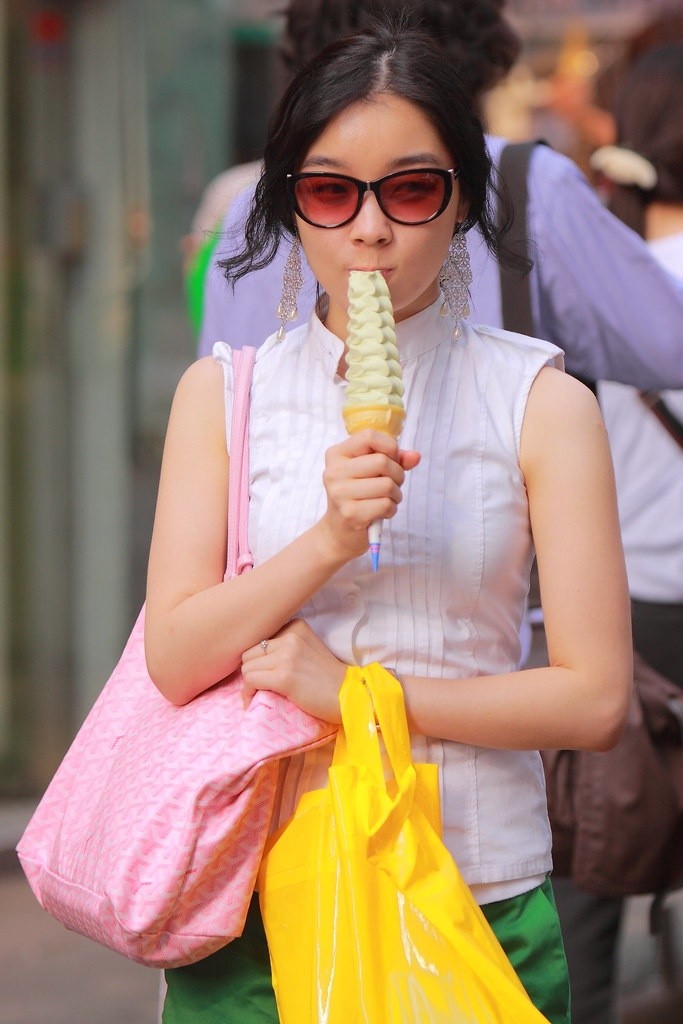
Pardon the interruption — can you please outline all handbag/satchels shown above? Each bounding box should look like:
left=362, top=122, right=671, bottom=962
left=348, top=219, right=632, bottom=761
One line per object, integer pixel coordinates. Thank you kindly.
left=539, top=653, right=683, bottom=895
left=16, top=347, right=348, bottom=967
left=260, top=665, right=558, bottom=1024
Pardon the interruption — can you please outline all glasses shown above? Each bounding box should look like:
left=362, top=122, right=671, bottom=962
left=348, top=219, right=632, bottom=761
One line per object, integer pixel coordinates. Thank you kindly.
left=285, top=168, right=458, bottom=229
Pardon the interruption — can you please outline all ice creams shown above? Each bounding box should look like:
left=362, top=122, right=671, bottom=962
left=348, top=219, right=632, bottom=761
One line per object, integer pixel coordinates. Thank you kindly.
left=343, top=271, right=406, bottom=437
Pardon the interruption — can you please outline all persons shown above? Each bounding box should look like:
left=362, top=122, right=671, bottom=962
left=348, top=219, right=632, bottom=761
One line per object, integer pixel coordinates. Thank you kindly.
left=143, top=25, right=632, bottom=1024
left=177, top=1, right=682, bottom=1024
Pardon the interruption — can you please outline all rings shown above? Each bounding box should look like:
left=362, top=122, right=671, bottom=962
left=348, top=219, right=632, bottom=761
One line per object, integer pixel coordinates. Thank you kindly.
left=262, top=640, right=269, bottom=655
